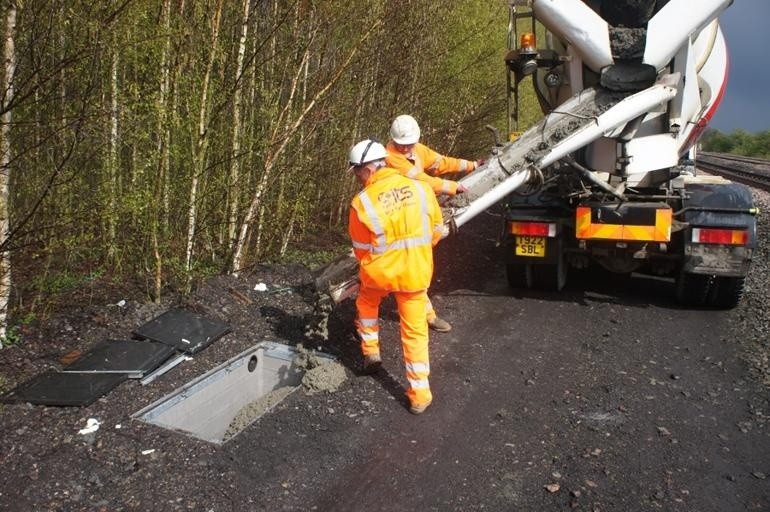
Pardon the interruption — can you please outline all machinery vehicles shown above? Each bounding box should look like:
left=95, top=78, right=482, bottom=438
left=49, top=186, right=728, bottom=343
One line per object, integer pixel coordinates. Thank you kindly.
left=317, top=1, right=759, bottom=317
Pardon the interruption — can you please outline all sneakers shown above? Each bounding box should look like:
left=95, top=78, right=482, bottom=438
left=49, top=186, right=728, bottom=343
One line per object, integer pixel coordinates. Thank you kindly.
left=428, top=317, right=452, bottom=332
left=410, top=399, right=431, bottom=414
left=364, top=352, right=382, bottom=374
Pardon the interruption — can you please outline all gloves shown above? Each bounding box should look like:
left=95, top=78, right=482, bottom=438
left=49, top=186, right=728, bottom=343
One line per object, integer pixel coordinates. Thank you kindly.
left=456, top=184, right=468, bottom=193
left=474, top=155, right=486, bottom=168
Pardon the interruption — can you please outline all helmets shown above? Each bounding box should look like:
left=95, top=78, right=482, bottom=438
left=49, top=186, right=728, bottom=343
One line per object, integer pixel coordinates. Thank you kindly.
left=390, top=115, right=420, bottom=144
left=346, top=140, right=388, bottom=172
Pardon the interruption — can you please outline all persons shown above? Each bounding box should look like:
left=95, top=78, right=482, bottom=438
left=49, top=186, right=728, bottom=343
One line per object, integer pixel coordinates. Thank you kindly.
left=347, top=139, right=444, bottom=415
left=381, top=114, right=485, bottom=332
